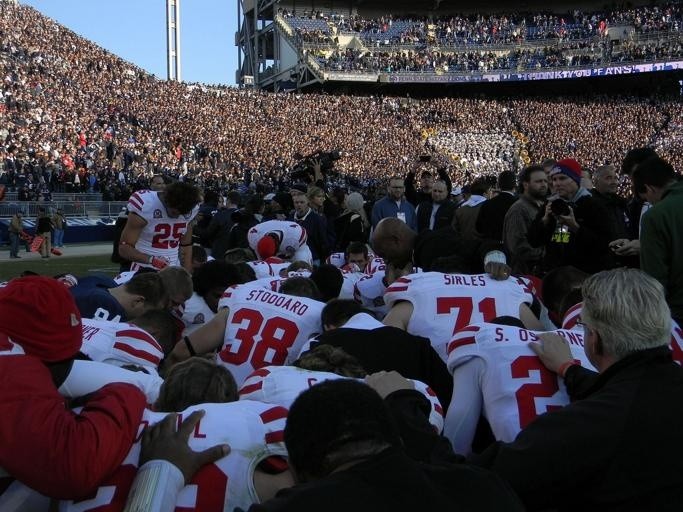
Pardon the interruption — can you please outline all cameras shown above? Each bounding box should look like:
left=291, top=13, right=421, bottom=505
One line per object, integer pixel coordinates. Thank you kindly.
left=419, top=155, right=432, bottom=163
left=550, top=196, right=577, bottom=219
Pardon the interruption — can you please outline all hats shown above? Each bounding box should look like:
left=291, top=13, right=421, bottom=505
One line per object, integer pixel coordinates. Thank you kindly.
left=549, top=158, right=583, bottom=188
left=256, top=235, right=276, bottom=260
left=290, top=183, right=308, bottom=193
left=345, top=192, right=364, bottom=212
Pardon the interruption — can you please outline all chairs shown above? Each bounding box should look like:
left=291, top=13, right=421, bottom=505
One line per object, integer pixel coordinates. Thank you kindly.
left=275, top=6, right=683, bottom=70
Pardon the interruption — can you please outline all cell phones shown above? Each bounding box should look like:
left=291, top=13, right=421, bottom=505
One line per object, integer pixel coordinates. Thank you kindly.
left=615, top=242, right=624, bottom=249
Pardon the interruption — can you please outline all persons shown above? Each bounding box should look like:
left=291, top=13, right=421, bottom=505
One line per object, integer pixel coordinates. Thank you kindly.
left=2, top=0, right=682, bottom=214
left=1, top=145, right=682, bottom=511
